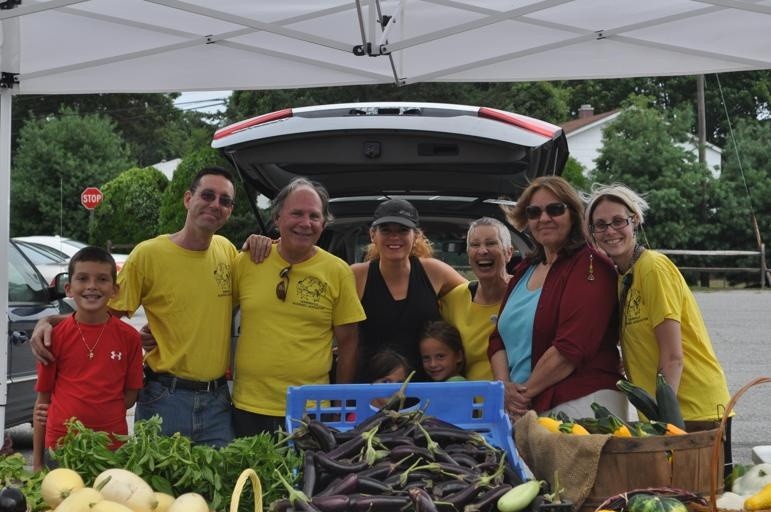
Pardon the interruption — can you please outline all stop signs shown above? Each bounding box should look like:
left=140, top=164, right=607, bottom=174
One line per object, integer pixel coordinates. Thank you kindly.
left=81, top=187, right=103, bottom=211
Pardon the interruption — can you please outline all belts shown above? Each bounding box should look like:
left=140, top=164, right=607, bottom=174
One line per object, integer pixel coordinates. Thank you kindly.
left=147, top=373, right=226, bottom=392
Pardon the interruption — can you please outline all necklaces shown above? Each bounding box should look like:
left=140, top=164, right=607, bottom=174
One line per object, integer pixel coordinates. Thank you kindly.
left=76, top=319, right=106, bottom=360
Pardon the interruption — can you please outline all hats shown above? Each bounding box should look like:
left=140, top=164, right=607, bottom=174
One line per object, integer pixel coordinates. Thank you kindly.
left=372, top=199, right=419, bottom=228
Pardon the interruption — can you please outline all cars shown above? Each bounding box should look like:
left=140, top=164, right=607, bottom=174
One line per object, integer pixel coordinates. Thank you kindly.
left=4, top=234, right=147, bottom=428
left=209, top=102, right=570, bottom=403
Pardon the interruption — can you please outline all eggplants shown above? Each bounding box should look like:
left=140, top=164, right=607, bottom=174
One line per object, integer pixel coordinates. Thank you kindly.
left=0, top=486, right=27, bottom=512
left=273, top=370, right=574, bottom=512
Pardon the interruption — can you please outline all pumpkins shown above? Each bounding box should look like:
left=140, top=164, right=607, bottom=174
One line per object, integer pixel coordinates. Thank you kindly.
left=626, top=493, right=688, bottom=512
left=536, top=374, right=688, bottom=439
left=745, top=483, right=771, bottom=511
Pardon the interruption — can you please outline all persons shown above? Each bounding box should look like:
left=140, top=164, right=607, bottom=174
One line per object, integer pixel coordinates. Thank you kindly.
left=29, top=165, right=272, bottom=451
left=272, top=198, right=468, bottom=384
left=345, top=341, right=410, bottom=422
left=138, top=175, right=370, bottom=445
left=438, top=215, right=514, bottom=380
left=486, top=176, right=629, bottom=422
left=582, top=181, right=736, bottom=492
left=418, top=320, right=466, bottom=381
left=33, top=246, right=144, bottom=472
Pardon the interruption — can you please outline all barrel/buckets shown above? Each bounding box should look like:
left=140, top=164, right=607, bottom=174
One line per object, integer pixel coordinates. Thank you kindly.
left=543, top=413, right=727, bottom=510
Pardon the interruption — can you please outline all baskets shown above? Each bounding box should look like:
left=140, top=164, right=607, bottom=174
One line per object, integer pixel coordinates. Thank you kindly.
left=282, top=381, right=525, bottom=493
left=597, top=488, right=707, bottom=512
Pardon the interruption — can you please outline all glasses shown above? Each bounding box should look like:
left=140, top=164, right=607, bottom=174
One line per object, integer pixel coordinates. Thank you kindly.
left=525, top=202, right=567, bottom=219
left=590, top=216, right=631, bottom=233
left=200, top=191, right=234, bottom=208
left=277, top=266, right=292, bottom=301
